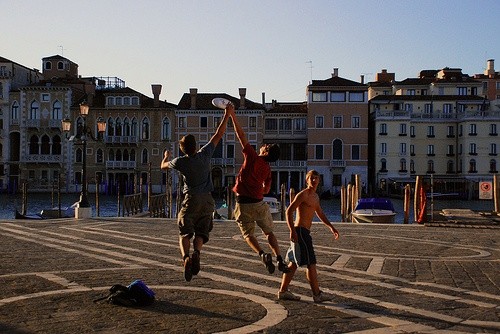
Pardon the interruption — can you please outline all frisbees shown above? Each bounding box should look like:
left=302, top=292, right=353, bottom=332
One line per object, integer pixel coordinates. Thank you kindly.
left=211, top=98, right=232, bottom=109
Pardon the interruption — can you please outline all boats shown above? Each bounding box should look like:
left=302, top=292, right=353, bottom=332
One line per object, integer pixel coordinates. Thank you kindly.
left=263, top=196, right=280, bottom=220
left=212, top=204, right=235, bottom=221
left=351, top=198, right=397, bottom=223
left=15, top=206, right=75, bottom=220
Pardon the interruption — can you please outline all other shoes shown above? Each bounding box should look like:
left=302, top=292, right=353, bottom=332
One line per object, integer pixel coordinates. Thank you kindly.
left=261, top=253, right=275, bottom=274
left=277, top=260, right=290, bottom=273
left=192, top=251, right=200, bottom=275
left=183, top=257, right=194, bottom=281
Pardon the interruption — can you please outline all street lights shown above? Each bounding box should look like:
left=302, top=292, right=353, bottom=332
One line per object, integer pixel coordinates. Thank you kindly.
left=60, top=98, right=107, bottom=208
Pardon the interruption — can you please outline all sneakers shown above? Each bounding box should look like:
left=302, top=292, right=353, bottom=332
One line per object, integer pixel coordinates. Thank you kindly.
left=313, top=291, right=337, bottom=303
left=278, top=290, right=301, bottom=300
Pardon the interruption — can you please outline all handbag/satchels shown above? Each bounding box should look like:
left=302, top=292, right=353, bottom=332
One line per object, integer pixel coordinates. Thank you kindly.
left=93, top=280, right=155, bottom=308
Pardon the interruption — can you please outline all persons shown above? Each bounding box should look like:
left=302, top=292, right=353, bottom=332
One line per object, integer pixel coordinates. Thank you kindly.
left=277, top=169, right=340, bottom=302
left=227, top=105, right=291, bottom=274
left=161, top=102, right=232, bottom=282
